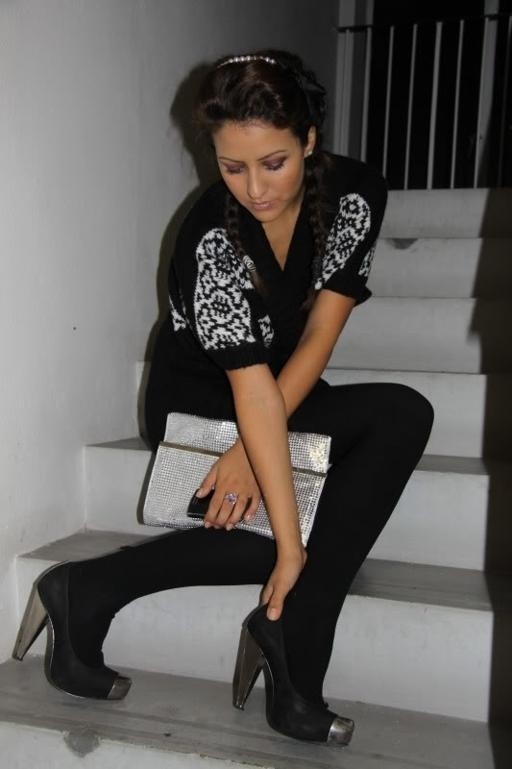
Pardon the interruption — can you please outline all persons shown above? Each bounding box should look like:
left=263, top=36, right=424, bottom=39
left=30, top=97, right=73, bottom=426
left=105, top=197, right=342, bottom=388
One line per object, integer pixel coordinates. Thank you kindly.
left=10, top=48, right=436, bottom=750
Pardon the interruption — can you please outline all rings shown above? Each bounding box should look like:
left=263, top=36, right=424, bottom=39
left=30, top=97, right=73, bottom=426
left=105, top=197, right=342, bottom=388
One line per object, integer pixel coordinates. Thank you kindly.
left=222, top=493, right=238, bottom=504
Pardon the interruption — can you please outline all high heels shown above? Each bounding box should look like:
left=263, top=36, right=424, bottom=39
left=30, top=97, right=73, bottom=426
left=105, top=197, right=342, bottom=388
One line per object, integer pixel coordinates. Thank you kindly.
left=11, top=560, right=131, bottom=699
left=232, top=602, right=355, bottom=743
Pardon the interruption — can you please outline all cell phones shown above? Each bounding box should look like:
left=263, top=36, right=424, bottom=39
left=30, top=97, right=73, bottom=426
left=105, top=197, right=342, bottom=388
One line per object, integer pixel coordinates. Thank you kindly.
left=187, top=488, right=253, bottom=522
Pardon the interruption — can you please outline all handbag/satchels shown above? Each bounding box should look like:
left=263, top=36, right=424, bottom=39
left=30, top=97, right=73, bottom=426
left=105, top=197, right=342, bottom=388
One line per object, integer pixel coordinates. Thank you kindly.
left=143, top=412, right=331, bottom=548
